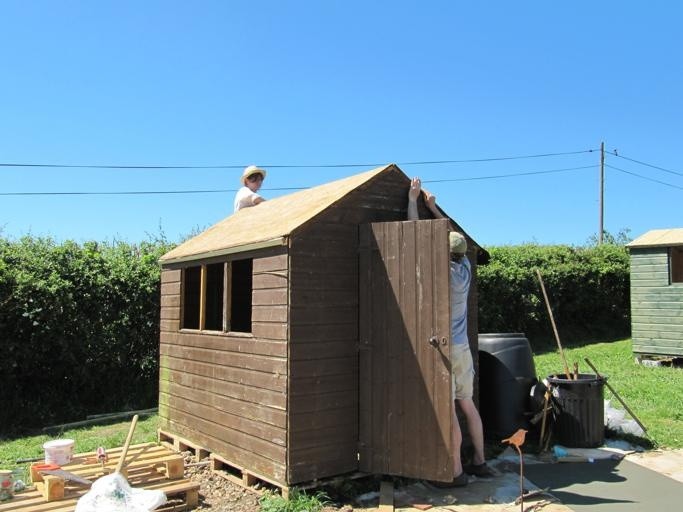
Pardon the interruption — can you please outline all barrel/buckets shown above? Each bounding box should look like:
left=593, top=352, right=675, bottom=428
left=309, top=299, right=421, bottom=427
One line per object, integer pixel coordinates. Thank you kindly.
left=476, top=332, right=538, bottom=441
left=43, top=439, right=75, bottom=466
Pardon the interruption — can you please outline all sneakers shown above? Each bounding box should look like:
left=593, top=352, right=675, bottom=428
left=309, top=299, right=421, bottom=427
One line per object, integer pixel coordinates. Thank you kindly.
left=430, top=462, right=496, bottom=488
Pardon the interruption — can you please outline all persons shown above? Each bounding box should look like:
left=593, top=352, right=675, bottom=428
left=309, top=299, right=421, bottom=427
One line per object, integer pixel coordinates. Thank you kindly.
left=234, top=163, right=265, bottom=210
left=406, top=175, right=494, bottom=490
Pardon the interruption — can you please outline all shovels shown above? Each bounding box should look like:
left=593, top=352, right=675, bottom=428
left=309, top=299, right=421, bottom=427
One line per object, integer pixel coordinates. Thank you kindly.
left=32, top=460, right=93, bottom=487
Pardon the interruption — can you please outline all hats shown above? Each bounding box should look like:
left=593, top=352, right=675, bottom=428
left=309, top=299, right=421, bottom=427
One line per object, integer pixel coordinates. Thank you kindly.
left=239, top=165, right=267, bottom=185
left=448, top=231, right=467, bottom=253
